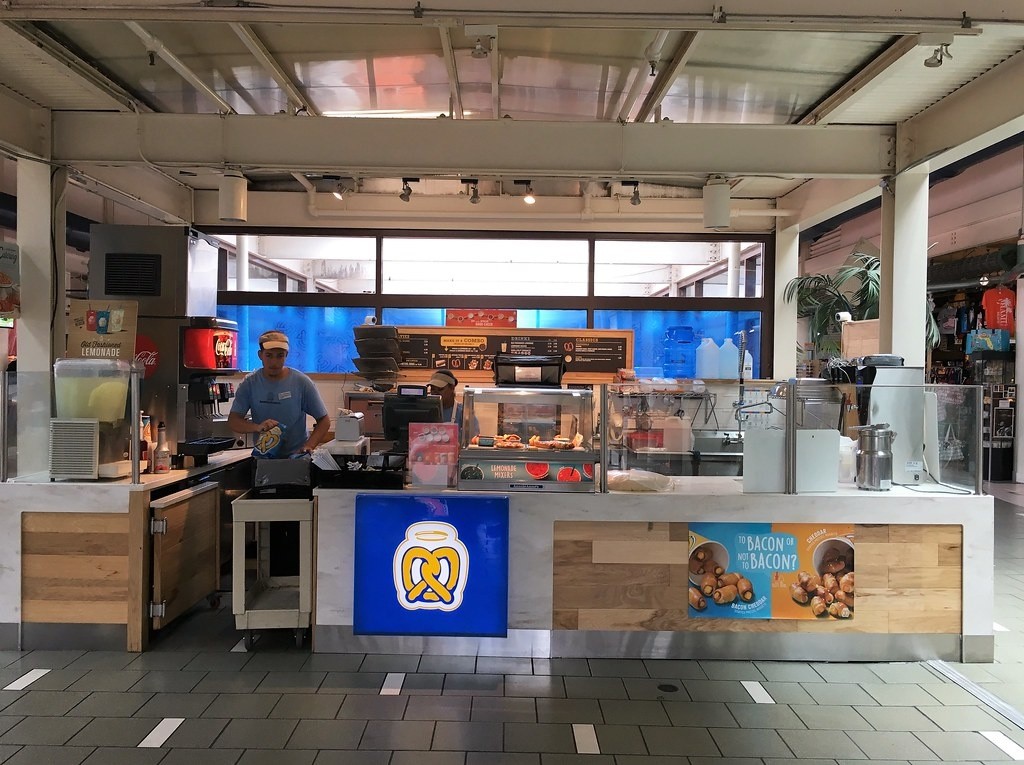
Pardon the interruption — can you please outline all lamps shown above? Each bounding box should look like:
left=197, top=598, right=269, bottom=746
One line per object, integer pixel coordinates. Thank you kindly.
left=701, top=173, right=731, bottom=227
left=630, top=185, right=641, bottom=205
left=924, top=43, right=954, bottom=68
left=524, top=184, right=535, bottom=203
left=218, top=168, right=247, bottom=221
left=332, top=183, right=350, bottom=200
left=399, top=182, right=412, bottom=202
left=469, top=185, right=481, bottom=205
left=472, top=36, right=494, bottom=58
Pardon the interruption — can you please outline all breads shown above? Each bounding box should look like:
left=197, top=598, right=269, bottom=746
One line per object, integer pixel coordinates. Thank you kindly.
left=688, top=546, right=756, bottom=611
left=790, top=548, right=855, bottom=619
left=471, top=433, right=575, bottom=450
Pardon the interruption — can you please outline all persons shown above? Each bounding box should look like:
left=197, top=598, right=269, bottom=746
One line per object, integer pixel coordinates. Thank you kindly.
left=426, top=369, right=480, bottom=444
left=227, top=330, right=331, bottom=458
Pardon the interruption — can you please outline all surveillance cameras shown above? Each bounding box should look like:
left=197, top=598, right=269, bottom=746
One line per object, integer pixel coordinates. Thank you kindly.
left=365, top=316, right=377, bottom=325
left=835, top=312, right=852, bottom=322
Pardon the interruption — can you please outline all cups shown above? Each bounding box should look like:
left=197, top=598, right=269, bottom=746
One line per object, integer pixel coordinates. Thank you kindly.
left=688, top=530, right=730, bottom=586
left=212, top=328, right=233, bottom=369
left=141, top=416, right=153, bottom=443
left=812, top=532, right=855, bottom=580
left=86, top=309, right=125, bottom=334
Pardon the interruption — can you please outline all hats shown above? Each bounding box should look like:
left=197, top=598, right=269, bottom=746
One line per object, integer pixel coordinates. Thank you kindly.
left=258, top=331, right=289, bottom=351
left=426, top=371, right=455, bottom=389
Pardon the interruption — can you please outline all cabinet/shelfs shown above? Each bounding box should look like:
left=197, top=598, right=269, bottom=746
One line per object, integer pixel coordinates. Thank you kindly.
left=230, top=486, right=313, bottom=648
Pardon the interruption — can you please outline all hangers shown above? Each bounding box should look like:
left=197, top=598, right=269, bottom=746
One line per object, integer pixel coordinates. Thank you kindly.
left=996, top=283, right=1004, bottom=288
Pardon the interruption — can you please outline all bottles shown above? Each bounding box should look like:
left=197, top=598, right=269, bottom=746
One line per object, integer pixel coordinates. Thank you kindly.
left=662, top=326, right=698, bottom=379
left=742, top=350, right=753, bottom=379
left=140, top=421, right=148, bottom=473
left=695, top=337, right=721, bottom=379
left=719, top=338, right=739, bottom=379
left=154, top=421, right=170, bottom=474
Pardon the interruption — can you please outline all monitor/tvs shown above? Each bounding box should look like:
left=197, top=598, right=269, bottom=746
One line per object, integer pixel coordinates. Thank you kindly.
left=382, top=393, right=444, bottom=455
